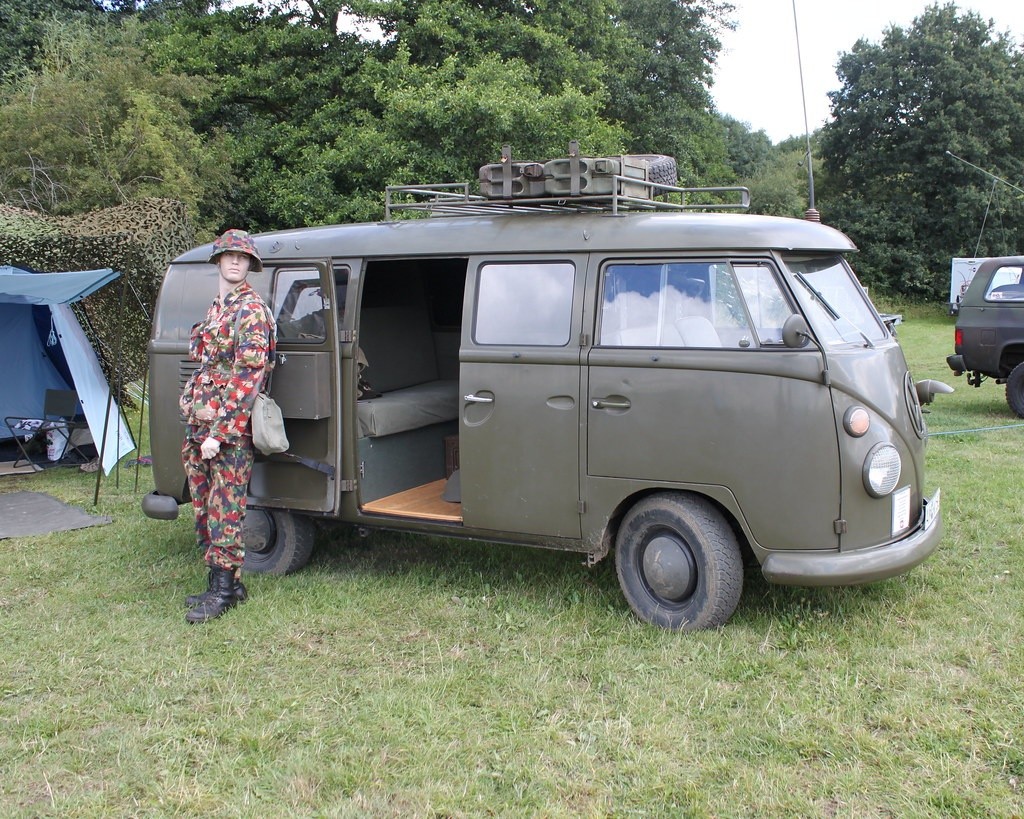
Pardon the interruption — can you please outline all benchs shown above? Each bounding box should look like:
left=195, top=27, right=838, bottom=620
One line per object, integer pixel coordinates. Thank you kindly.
left=335, top=306, right=459, bottom=437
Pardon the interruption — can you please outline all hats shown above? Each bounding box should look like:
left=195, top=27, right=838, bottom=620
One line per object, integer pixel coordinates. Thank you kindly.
left=207, top=228, right=264, bottom=273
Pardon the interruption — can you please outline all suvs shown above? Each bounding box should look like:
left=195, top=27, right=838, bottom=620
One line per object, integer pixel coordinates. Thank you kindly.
left=946, top=255, right=1024, bottom=420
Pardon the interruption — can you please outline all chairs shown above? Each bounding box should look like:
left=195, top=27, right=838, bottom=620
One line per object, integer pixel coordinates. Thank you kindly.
left=4, top=389, right=90, bottom=473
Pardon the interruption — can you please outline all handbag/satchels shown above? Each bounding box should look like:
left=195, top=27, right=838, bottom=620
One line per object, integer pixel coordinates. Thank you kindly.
left=251, top=392, right=289, bottom=456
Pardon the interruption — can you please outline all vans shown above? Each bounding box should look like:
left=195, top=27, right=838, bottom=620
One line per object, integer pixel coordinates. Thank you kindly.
left=139, top=146, right=953, bottom=631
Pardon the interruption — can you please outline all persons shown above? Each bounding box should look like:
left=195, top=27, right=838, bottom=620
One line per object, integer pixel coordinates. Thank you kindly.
left=178, top=229, right=277, bottom=623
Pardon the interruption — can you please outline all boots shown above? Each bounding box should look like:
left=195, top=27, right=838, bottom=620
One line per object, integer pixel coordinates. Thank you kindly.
left=183, top=563, right=248, bottom=622
